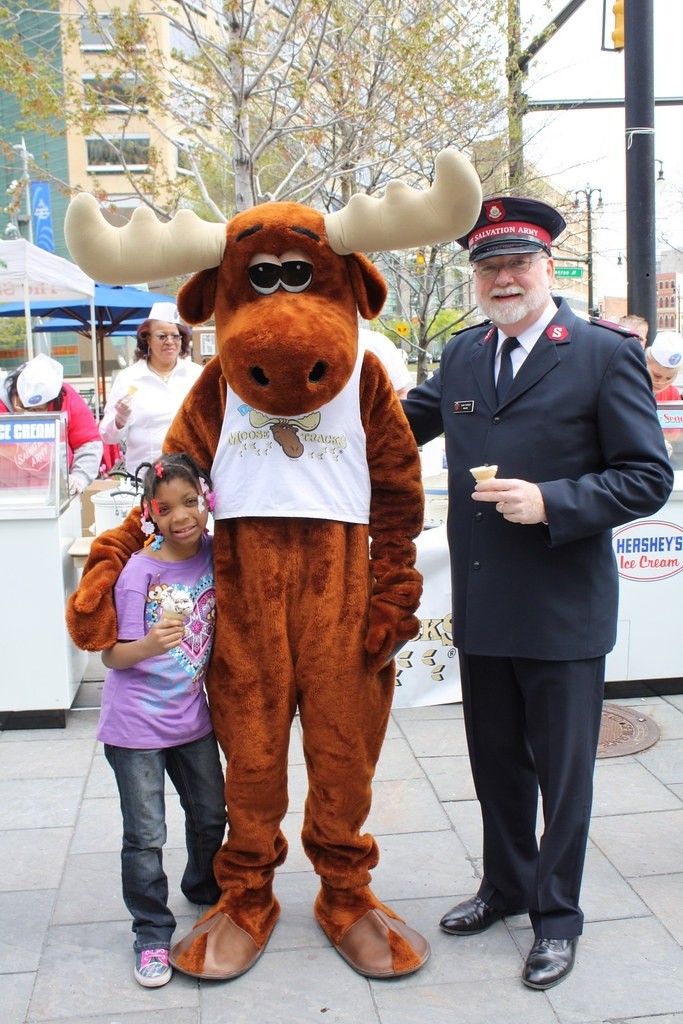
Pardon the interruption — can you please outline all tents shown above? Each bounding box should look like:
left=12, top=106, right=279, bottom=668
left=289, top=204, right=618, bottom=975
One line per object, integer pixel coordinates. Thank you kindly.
left=0, top=238, right=101, bottom=425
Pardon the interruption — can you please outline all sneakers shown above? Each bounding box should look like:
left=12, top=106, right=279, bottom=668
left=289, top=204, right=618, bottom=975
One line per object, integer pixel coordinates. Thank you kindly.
left=134, top=945, right=173, bottom=987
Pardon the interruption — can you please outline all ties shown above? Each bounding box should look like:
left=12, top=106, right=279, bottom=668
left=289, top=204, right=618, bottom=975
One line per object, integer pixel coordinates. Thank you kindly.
left=496, top=337, right=521, bottom=414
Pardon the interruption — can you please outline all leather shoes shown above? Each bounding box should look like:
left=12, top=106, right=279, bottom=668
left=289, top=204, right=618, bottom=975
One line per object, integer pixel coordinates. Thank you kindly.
left=521, top=936, right=579, bottom=989
left=439, top=895, right=530, bottom=936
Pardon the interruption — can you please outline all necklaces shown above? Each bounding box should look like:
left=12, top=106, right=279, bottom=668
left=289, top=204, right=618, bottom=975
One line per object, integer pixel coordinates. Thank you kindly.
left=149, top=361, right=177, bottom=383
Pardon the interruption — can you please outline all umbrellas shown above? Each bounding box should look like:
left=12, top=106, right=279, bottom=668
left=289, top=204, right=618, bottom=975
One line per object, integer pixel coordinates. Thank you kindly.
left=0, top=284, right=192, bottom=409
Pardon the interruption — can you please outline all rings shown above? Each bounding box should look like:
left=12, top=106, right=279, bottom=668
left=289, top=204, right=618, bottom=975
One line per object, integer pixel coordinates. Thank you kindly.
left=498, top=501, right=506, bottom=513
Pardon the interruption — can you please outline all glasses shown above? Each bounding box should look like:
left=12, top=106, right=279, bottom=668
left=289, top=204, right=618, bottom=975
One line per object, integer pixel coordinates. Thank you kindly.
left=148, top=332, right=182, bottom=342
left=473, top=255, right=550, bottom=279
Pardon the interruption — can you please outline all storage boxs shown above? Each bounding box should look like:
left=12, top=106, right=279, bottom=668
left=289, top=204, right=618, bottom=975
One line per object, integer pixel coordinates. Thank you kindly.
left=80, top=474, right=126, bottom=536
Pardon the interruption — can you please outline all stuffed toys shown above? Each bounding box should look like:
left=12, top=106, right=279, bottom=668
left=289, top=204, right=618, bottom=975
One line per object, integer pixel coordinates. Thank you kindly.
left=64, top=142, right=485, bottom=984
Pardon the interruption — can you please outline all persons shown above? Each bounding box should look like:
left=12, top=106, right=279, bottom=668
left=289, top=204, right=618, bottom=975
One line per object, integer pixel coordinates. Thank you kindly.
left=620, top=314, right=683, bottom=403
left=0, top=352, right=104, bottom=499
left=97, top=454, right=229, bottom=988
left=99, top=302, right=206, bottom=490
left=400, top=196, right=675, bottom=991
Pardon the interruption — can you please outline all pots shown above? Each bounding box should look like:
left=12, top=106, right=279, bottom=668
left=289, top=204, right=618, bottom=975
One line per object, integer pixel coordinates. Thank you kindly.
left=89, top=477, right=145, bottom=537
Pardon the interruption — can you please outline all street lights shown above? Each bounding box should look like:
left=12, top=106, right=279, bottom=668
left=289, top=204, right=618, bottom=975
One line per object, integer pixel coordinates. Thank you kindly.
left=12, top=136, right=33, bottom=362
left=573, top=183, right=604, bottom=320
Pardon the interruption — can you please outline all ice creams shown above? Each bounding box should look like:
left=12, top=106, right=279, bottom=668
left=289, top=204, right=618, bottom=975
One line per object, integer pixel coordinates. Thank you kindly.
left=129, top=385, right=138, bottom=395
left=470, top=463, right=498, bottom=484
left=160, top=589, right=194, bottom=621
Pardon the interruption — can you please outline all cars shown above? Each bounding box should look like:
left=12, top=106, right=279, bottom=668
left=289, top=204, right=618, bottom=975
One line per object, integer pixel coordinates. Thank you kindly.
left=426, top=354, right=442, bottom=364
left=407, top=352, right=418, bottom=363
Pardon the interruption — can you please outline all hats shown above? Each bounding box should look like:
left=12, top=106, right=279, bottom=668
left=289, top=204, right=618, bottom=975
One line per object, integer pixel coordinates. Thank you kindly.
left=454, top=196, right=567, bottom=264
left=16, top=352, right=62, bottom=408
left=651, top=331, right=683, bottom=368
left=148, top=302, right=179, bottom=326
